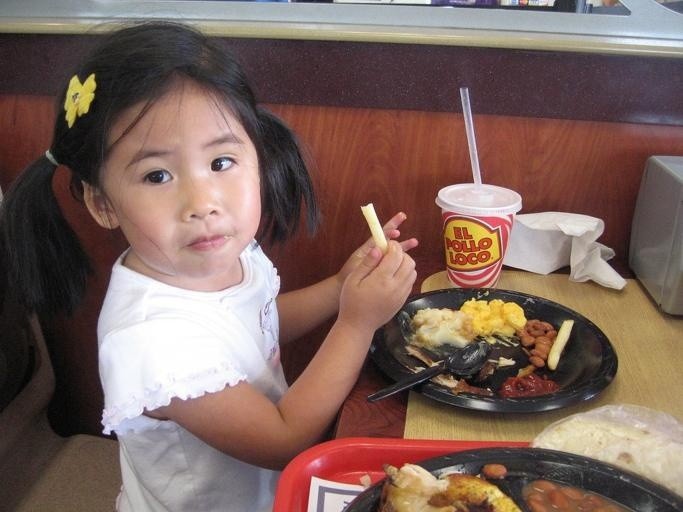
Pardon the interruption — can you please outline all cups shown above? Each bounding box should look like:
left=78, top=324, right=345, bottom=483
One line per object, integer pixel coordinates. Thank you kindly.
left=434, top=182, right=521, bottom=291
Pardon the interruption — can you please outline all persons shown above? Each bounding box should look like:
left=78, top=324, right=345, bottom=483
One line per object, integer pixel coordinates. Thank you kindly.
left=0, top=22, right=418, bottom=512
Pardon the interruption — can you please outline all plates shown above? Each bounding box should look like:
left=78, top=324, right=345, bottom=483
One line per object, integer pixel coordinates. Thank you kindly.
left=338, top=444, right=682, bottom=512
left=370, top=287, right=618, bottom=415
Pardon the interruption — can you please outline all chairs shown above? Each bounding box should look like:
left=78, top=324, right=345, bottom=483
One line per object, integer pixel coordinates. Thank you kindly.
left=0, top=184, right=122, bottom=512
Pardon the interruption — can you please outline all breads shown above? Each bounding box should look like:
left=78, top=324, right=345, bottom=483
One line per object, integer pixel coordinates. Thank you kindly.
left=562, top=413, right=683, bottom=499
left=380, top=463, right=522, bottom=512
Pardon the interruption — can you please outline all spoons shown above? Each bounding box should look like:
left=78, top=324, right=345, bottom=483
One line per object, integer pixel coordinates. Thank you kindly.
left=366, top=338, right=489, bottom=409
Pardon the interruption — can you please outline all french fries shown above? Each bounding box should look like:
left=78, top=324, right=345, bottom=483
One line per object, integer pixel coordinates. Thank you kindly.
left=360, top=203, right=387, bottom=248
left=548, top=319, right=575, bottom=371
